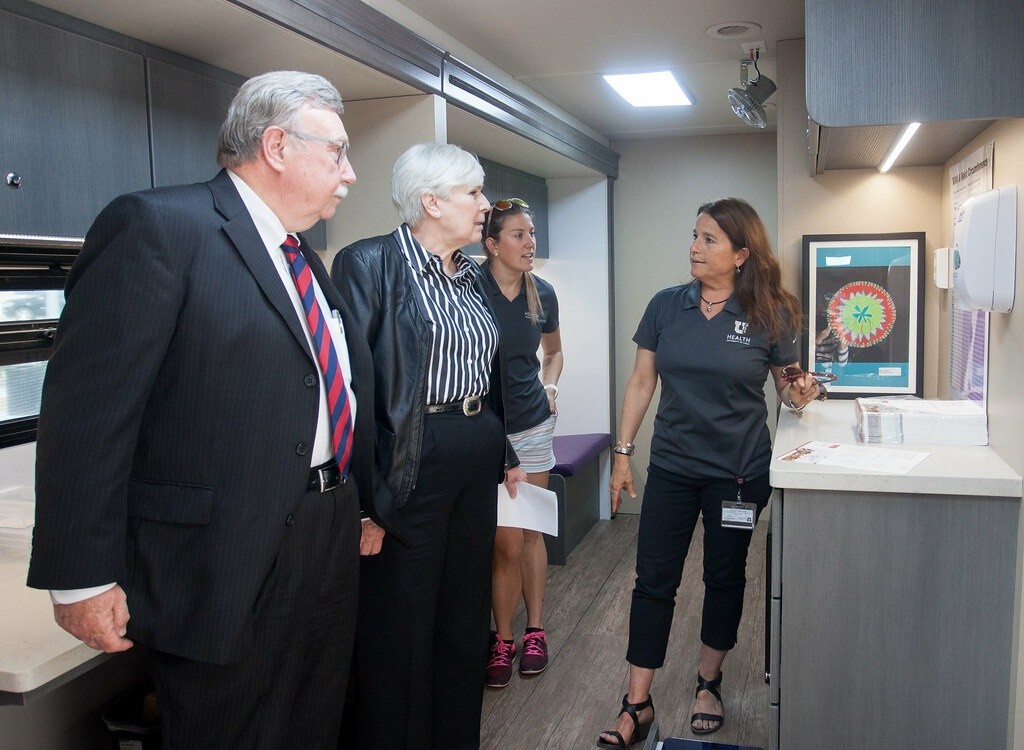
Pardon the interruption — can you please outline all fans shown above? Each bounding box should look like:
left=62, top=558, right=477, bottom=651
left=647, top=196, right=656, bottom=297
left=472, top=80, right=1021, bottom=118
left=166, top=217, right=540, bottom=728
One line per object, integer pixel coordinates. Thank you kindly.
left=726, top=73, right=777, bottom=129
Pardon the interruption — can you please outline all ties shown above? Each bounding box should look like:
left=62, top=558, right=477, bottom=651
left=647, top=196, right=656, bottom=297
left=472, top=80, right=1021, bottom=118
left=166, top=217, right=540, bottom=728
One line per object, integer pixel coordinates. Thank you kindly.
left=280, top=234, right=354, bottom=482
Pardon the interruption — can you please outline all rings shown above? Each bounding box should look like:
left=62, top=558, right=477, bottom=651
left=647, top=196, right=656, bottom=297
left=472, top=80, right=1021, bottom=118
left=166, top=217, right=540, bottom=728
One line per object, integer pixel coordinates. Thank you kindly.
left=609, top=490, right=613, bottom=494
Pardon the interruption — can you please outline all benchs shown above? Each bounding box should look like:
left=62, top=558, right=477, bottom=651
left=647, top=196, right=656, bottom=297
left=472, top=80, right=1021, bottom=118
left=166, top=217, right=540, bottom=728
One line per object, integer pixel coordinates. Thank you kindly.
left=544, top=431, right=611, bottom=569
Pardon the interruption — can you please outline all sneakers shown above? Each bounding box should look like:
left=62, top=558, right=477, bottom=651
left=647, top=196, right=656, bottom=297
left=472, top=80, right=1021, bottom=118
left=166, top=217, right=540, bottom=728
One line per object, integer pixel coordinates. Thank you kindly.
left=520, top=631, right=549, bottom=674
left=485, top=634, right=516, bottom=688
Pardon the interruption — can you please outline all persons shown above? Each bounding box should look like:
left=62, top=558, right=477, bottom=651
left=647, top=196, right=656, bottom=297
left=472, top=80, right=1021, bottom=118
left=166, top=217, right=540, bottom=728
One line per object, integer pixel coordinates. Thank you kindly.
left=330, top=141, right=527, bottom=750
left=595, top=197, right=822, bottom=750
left=815, top=292, right=848, bottom=385
left=481, top=198, right=564, bottom=687
left=28, top=70, right=376, bottom=750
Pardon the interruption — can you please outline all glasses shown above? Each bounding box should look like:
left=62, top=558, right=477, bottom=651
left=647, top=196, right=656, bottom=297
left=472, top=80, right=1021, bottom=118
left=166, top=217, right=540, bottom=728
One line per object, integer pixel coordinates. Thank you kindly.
left=283, top=130, right=348, bottom=163
left=486, top=198, right=529, bottom=238
left=780, top=365, right=836, bottom=387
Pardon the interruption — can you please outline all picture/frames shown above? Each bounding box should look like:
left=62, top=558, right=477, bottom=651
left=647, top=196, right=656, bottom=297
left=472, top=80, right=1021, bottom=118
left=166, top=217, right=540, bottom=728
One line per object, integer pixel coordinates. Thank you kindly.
left=801, top=231, right=927, bottom=400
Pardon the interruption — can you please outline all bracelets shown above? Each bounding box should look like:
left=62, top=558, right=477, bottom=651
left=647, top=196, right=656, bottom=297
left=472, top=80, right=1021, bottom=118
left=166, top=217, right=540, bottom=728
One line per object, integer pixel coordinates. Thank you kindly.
left=614, top=441, right=635, bottom=456
left=790, top=400, right=806, bottom=412
left=543, top=384, right=558, bottom=400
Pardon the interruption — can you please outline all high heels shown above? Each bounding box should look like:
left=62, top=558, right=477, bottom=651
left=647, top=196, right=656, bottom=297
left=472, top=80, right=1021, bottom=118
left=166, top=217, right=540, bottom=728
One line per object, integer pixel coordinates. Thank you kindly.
left=596, top=694, right=655, bottom=750
left=691, top=670, right=723, bottom=734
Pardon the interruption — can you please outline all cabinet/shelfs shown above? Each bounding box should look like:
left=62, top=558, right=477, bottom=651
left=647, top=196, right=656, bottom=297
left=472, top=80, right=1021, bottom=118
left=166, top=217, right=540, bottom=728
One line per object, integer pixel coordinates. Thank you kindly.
left=762, top=390, right=1023, bottom=750
left=0, top=1, right=328, bottom=253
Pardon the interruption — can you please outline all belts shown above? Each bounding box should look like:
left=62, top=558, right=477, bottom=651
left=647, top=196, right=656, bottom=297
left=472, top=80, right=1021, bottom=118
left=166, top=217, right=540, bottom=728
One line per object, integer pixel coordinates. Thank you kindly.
left=424, top=395, right=486, bottom=416
left=307, top=464, right=344, bottom=494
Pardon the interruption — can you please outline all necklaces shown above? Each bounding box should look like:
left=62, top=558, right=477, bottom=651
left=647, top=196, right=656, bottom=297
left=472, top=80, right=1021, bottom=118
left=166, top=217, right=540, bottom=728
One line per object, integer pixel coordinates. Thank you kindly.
left=701, top=295, right=728, bottom=312
